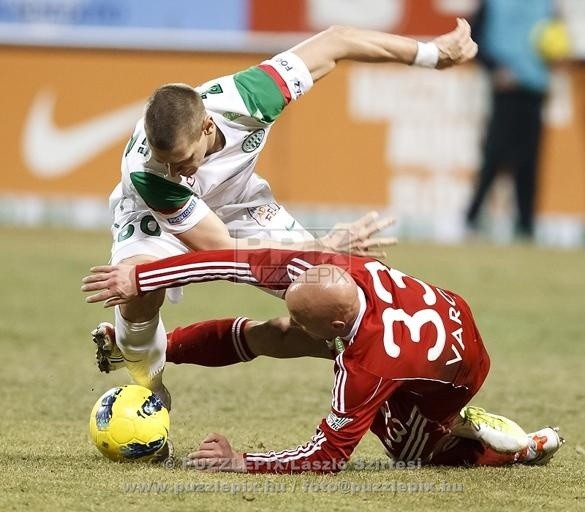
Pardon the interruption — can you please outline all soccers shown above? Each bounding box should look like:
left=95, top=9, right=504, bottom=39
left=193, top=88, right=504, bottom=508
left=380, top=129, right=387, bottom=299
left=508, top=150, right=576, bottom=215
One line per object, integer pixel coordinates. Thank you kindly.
left=89, top=384, right=169, bottom=463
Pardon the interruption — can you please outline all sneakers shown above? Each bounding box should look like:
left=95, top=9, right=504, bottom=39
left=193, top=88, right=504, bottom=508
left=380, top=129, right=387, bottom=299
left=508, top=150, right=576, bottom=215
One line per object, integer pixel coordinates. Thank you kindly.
left=446, top=406, right=528, bottom=454
left=91, top=321, right=126, bottom=374
left=153, top=385, right=175, bottom=462
left=517, top=426, right=567, bottom=466
left=166, top=285, right=184, bottom=303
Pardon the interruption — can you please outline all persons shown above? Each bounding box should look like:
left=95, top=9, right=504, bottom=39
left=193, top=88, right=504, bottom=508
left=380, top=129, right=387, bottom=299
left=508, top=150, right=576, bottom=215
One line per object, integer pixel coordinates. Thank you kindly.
left=457, top=0, right=564, bottom=248
left=82, top=246, right=567, bottom=475
left=105, top=15, right=531, bottom=463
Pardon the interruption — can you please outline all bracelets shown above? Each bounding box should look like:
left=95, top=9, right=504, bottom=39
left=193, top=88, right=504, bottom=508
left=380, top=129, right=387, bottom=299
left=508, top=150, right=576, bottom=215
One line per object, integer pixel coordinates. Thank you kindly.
left=412, top=43, right=441, bottom=70
